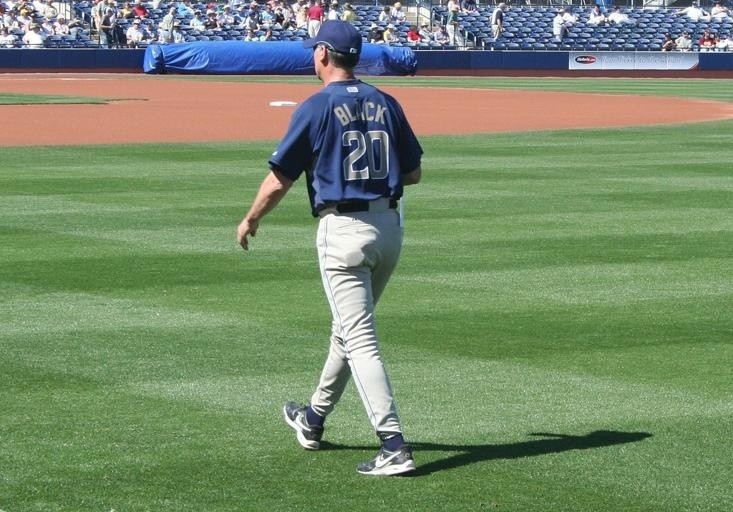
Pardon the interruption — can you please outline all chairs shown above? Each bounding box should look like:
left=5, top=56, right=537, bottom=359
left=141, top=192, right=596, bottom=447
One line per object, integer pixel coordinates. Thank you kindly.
left=0, top=0, right=733, bottom=55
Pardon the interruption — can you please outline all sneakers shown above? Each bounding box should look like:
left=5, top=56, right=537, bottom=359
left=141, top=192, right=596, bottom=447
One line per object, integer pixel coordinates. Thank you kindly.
left=356, top=444, right=416, bottom=477
left=283, top=401, right=324, bottom=451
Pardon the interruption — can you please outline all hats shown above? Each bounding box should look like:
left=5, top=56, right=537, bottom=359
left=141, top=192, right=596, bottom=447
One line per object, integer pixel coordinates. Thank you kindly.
left=303, top=20, right=362, bottom=54
left=98, top=0, right=398, bottom=31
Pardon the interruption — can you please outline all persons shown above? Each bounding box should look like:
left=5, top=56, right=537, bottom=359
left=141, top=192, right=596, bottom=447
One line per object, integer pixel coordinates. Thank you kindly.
left=553, top=4, right=629, bottom=41
left=0, top=0, right=506, bottom=48
left=662, top=0, right=733, bottom=51
left=238, top=20, right=424, bottom=476
